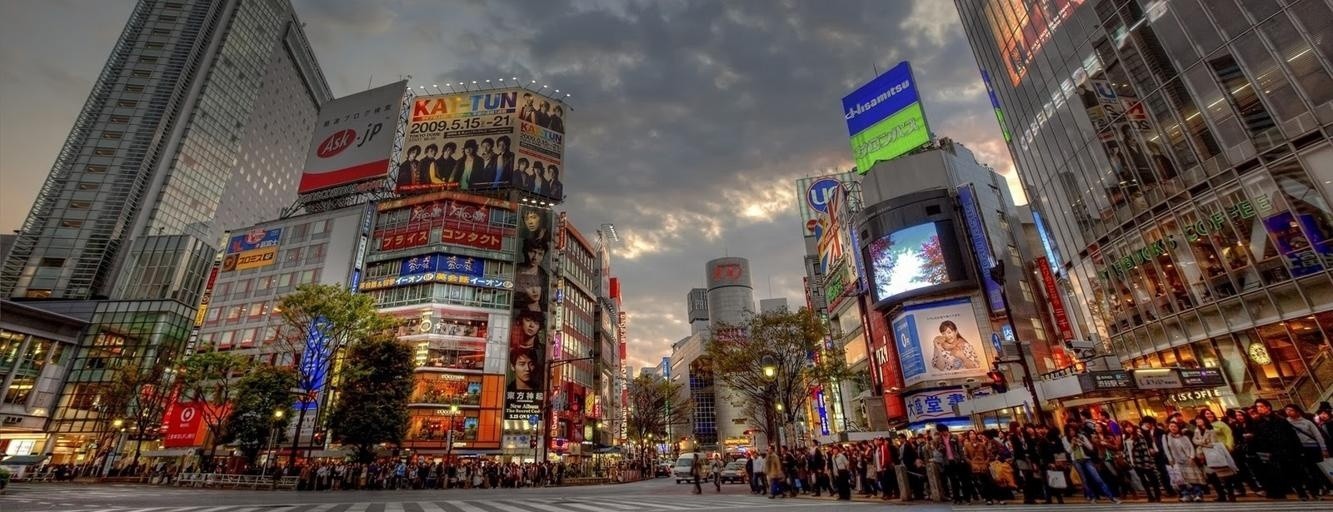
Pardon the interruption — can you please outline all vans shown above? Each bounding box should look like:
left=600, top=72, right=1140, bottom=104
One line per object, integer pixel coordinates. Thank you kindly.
left=673, top=452, right=712, bottom=484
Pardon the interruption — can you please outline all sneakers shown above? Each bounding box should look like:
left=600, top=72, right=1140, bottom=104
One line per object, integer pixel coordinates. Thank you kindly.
left=694, top=489, right=1332, bottom=505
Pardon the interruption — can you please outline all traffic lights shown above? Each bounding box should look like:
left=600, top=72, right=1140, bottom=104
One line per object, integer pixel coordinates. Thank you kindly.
left=529, top=438, right=538, bottom=450
left=987, top=368, right=1010, bottom=394
left=313, top=431, right=324, bottom=446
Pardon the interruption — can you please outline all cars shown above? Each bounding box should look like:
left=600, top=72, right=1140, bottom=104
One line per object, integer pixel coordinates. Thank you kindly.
left=708, top=458, right=750, bottom=484
left=655, top=465, right=672, bottom=478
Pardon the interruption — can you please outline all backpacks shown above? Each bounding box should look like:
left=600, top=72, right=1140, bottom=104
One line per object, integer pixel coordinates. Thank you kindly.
left=1202, top=442, right=1228, bottom=468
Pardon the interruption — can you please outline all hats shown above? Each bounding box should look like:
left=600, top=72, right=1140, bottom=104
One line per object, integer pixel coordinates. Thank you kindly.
left=461, top=139, right=480, bottom=150
left=531, top=161, right=544, bottom=168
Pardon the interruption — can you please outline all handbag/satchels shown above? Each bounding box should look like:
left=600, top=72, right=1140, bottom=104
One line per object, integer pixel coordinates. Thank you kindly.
left=1316, top=456, right=1333, bottom=483
left=1068, top=463, right=1089, bottom=490
left=1111, top=451, right=1131, bottom=471
left=1082, top=445, right=1090, bottom=455
left=1046, top=466, right=1068, bottom=489
left=770, top=479, right=783, bottom=495
left=865, top=462, right=878, bottom=481
left=837, top=470, right=848, bottom=479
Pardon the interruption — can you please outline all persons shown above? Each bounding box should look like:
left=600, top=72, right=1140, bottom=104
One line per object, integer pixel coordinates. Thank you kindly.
left=516, top=239, right=547, bottom=275
left=518, top=91, right=535, bottom=123
left=510, top=310, right=544, bottom=349
left=530, top=100, right=550, bottom=128
left=519, top=206, right=550, bottom=241
left=506, top=348, right=543, bottom=390
left=396, top=134, right=561, bottom=201
left=929, top=320, right=979, bottom=368
left=548, top=105, right=562, bottom=132
left=514, top=274, right=547, bottom=312
left=38, top=396, right=1333, bottom=504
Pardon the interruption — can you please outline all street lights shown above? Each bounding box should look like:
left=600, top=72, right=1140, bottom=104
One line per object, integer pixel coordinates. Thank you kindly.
left=445, top=400, right=457, bottom=467
left=760, top=355, right=788, bottom=457
left=94, top=418, right=128, bottom=479
left=259, top=408, right=284, bottom=479
